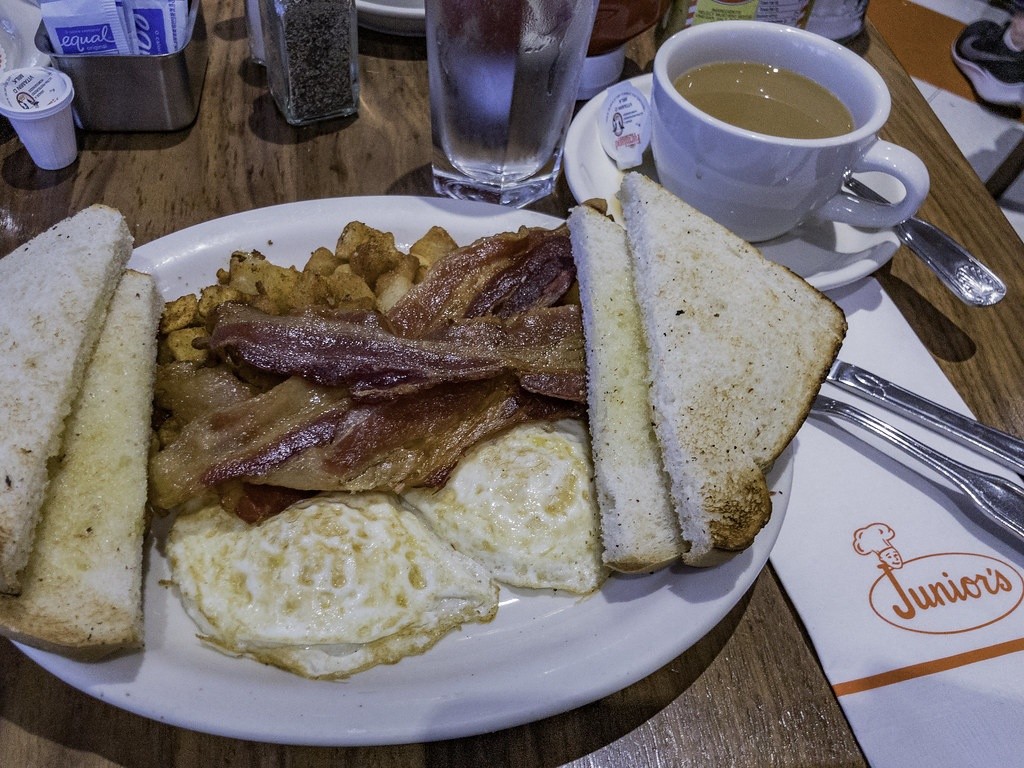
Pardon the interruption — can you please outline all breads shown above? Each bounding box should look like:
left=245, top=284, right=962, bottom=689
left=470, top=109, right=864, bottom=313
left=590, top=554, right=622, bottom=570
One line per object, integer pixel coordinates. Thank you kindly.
left=0, top=203, right=134, bottom=597
left=621, top=170, right=848, bottom=569
left=0, top=269, right=166, bottom=661
left=568, top=207, right=694, bottom=577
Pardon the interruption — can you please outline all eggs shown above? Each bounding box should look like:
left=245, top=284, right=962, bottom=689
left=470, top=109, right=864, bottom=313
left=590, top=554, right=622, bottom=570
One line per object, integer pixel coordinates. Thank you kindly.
left=161, top=488, right=502, bottom=679
left=399, top=417, right=615, bottom=595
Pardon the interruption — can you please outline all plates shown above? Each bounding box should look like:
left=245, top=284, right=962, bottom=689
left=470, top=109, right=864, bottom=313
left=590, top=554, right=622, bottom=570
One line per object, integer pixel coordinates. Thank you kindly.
left=563, top=73, right=916, bottom=292
left=354, top=0, right=427, bottom=37
left=7, top=195, right=795, bottom=745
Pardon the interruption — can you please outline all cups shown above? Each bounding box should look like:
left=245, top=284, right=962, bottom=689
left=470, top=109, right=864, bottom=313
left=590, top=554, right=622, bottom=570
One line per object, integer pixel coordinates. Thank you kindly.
left=425, top=0, right=600, bottom=210
left=0, top=67, right=79, bottom=171
left=649, top=19, right=930, bottom=242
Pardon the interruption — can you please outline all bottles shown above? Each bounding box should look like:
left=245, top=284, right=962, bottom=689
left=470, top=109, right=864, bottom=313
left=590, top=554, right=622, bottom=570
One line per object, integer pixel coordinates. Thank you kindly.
left=244, top=0, right=359, bottom=124
